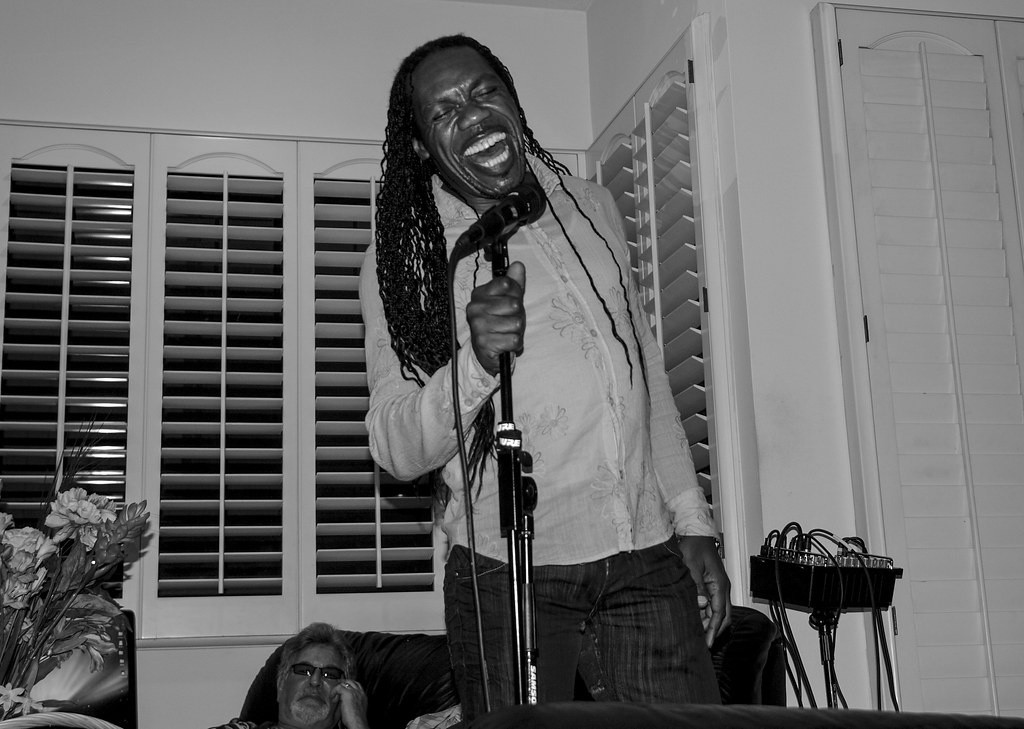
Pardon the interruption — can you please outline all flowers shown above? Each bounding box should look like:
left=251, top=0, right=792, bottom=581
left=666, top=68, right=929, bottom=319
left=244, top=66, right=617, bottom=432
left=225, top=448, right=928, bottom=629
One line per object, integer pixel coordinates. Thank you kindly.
left=0, top=420, right=150, bottom=721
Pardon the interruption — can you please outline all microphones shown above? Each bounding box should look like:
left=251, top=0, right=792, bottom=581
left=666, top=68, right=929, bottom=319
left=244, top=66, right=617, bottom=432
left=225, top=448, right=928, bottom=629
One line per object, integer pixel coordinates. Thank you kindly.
left=457, top=183, right=546, bottom=251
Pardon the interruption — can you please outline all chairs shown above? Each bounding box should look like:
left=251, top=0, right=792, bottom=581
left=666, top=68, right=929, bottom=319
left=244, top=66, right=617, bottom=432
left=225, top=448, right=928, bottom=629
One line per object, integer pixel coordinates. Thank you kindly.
left=239, top=631, right=459, bottom=729
left=575, top=604, right=786, bottom=708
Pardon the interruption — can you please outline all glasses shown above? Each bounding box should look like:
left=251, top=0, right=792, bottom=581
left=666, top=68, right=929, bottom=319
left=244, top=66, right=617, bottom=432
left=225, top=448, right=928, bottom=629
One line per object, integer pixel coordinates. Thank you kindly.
left=283, top=664, right=347, bottom=682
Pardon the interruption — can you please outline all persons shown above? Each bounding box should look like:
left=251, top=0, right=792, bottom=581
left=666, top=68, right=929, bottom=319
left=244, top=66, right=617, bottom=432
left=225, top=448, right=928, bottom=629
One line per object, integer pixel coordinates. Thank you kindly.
left=357, top=35, right=734, bottom=720
left=208, top=621, right=368, bottom=729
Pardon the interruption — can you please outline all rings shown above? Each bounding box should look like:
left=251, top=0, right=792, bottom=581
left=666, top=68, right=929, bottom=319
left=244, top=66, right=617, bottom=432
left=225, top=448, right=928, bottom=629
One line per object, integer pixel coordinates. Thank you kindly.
left=346, top=682, right=350, bottom=689
